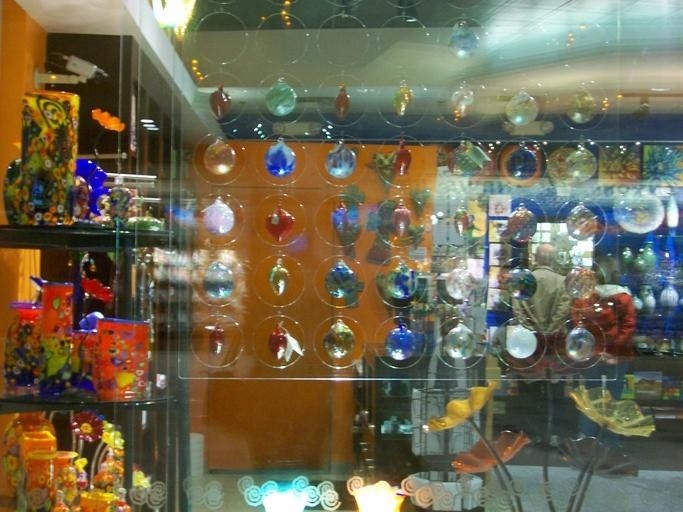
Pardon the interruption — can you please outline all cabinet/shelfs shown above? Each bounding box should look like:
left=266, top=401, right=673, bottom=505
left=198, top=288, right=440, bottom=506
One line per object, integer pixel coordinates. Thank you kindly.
left=355, top=345, right=489, bottom=510
left=1, top=0, right=176, bottom=512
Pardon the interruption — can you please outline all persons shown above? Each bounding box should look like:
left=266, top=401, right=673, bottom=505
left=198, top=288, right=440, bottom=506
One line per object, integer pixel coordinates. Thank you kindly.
left=511, top=244, right=572, bottom=447
left=564, top=264, right=637, bottom=459
